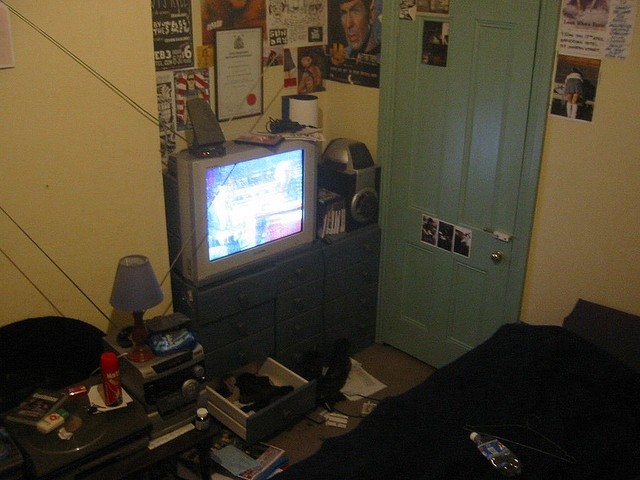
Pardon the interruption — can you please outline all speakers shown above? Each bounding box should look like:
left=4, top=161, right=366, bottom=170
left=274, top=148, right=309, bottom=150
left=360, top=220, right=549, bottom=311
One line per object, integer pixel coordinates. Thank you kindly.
left=317, top=137, right=381, bottom=233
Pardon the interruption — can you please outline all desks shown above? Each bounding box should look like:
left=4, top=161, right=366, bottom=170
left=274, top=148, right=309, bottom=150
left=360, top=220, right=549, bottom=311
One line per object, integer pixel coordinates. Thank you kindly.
left=0, top=379, right=222, bottom=478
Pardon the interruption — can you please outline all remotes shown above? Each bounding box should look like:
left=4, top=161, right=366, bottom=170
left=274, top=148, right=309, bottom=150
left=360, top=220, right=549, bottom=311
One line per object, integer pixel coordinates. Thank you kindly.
left=305, top=408, right=327, bottom=425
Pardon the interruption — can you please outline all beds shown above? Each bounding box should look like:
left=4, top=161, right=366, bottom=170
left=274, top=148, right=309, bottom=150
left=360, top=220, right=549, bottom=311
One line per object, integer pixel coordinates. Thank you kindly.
left=257, top=296, right=640, bottom=478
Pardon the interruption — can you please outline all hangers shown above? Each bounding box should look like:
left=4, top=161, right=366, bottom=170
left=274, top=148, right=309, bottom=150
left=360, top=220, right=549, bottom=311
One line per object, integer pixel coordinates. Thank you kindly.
left=461, top=415, right=578, bottom=464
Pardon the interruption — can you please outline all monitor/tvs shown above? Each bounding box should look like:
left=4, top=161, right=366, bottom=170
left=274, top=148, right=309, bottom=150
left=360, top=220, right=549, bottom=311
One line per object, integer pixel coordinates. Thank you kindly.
left=162, top=139, right=321, bottom=289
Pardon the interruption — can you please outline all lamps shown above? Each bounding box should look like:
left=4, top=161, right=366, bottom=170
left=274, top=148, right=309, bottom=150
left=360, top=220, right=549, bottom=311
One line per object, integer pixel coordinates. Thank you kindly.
left=110, top=255, right=164, bottom=361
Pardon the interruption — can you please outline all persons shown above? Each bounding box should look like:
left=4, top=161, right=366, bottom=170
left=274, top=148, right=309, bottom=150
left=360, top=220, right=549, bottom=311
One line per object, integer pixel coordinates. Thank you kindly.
left=564, top=72, right=584, bottom=119
left=298, top=55, right=321, bottom=94
left=329, top=0, right=382, bottom=68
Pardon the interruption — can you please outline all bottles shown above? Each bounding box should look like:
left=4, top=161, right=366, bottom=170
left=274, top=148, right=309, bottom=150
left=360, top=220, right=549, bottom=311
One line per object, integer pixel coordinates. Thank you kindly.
left=469, top=431, right=524, bottom=479
left=100, top=351, right=123, bottom=407
left=195, top=407, right=211, bottom=432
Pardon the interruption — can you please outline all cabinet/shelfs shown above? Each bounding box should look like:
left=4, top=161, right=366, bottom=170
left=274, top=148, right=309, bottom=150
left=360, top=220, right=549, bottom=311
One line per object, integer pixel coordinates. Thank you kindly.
left=174, top=229, right=383, bottom=444
